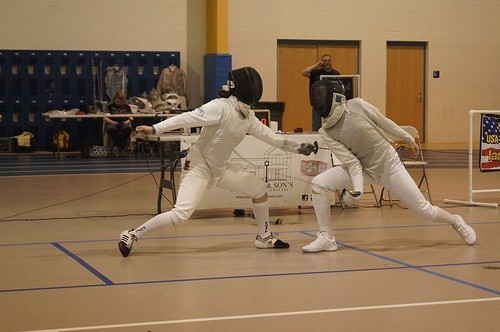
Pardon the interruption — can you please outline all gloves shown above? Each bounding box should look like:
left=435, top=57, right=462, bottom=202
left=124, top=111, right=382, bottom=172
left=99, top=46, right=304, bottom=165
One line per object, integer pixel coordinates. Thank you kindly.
left=299, top=142, right=315, bottom=156
left=342, top=175, right=364, bottom=205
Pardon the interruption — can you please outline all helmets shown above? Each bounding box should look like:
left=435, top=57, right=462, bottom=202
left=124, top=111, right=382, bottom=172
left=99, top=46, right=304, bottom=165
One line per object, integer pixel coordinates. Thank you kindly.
left=228, top=66, right=263, bottom=105
left=310, top=79, right=346, bottom=129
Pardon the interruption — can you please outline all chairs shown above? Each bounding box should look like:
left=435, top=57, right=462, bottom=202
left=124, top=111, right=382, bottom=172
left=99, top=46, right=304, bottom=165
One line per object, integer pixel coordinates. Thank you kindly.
left=379, top=125, right=434, bottom=207
left=332, top=153, right=379, bottom=210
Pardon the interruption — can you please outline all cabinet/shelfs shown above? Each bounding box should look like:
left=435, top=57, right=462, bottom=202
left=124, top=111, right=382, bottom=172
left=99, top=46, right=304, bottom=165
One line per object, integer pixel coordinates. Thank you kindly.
left=0, top=50, right=181, bottom=150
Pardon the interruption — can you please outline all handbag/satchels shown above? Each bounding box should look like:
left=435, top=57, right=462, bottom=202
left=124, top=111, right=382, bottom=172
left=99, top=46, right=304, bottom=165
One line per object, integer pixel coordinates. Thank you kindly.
left=88, top=143, right=111, bottom=158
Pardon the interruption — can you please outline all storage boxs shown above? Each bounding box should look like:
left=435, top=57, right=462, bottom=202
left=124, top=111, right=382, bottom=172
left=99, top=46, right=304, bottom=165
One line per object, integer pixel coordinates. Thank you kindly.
left=81, top=144, right=107, bottom=158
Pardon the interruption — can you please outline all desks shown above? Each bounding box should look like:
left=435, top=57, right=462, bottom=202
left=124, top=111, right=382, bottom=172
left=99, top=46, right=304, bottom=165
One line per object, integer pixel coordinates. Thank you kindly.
left=42, top=111, right=191, bottom=161
left=135, top=133, right=335, bottom=214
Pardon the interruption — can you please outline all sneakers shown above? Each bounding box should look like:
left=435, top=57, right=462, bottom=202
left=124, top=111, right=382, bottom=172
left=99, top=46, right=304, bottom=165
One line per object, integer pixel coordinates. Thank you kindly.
left=302, top=237, right=339, bottom=254
left=254, top=234, right=291, bottom=251
left=118, top=230, right=135, bottom=257
left=451, top=215, right=477, bottom=246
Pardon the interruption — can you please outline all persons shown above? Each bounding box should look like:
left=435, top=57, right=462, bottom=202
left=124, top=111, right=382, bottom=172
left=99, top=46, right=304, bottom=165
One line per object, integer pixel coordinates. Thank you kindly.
left=302, top=78, right=476, bottom=253
left=300, top=54, right=340, bottom=131
left=155, top=62, right=189, bottom=107
left=118, top=67, right=316, bottom=258
left=104, top=91, right=134, bottom=158
